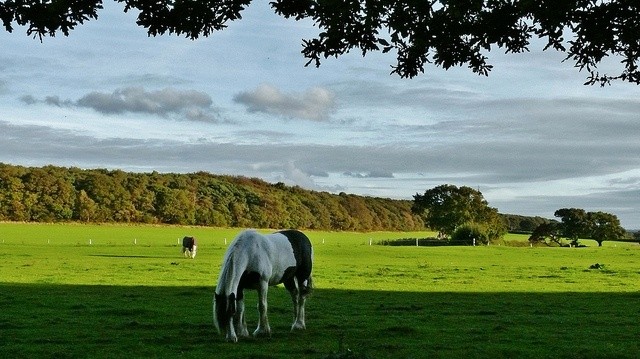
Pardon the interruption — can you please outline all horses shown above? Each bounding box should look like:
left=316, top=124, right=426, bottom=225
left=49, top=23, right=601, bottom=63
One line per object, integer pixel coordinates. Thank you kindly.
left=211, top=229, right=316, bottom=345
left=181, top=235, right=197, bottom=260
